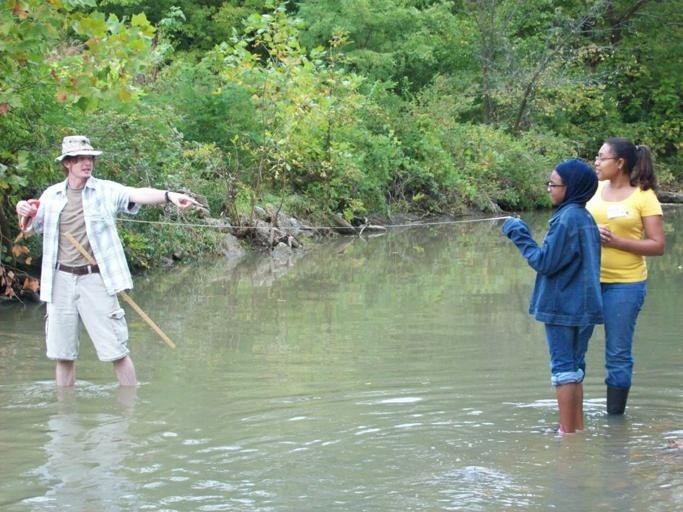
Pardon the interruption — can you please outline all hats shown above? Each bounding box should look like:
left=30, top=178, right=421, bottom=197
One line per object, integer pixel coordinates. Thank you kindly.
left=53, top=134, right=103, bottom=164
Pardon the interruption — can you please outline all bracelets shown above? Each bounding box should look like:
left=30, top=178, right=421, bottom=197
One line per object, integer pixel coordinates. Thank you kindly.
left=164, top=191, right=170, bottom=204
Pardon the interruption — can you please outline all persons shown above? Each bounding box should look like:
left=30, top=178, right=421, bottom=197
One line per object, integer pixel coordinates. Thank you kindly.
left=583, top=136, right=666, bottom=416
left=500, top=160, right=604, bottom=437
left=15, top=135, right=204, bottom=391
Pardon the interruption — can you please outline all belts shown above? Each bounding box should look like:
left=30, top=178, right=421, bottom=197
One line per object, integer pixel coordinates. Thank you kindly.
left=53, top=261, right=99, bottom=275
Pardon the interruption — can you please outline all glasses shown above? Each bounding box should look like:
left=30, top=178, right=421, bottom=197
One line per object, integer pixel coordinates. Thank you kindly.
left=593, top=154, right=618, bottom=164
left=547, top=179, right=565, bottom=191
